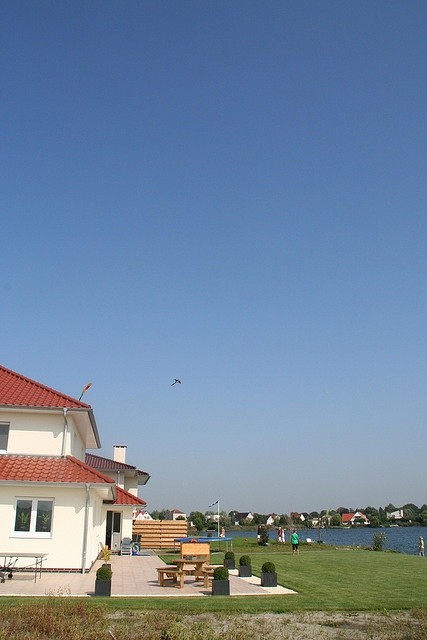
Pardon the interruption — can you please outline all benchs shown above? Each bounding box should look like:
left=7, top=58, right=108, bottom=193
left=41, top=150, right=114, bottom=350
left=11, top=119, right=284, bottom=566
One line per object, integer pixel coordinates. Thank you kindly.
left=157, top=569, right=184, bottom=589
left=199, top=568, right=220, bottom=589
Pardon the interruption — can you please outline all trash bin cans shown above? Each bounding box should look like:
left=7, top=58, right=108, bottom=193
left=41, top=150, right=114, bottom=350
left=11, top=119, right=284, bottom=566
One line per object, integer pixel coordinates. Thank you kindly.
left=132, top=535, right=142, bottom=551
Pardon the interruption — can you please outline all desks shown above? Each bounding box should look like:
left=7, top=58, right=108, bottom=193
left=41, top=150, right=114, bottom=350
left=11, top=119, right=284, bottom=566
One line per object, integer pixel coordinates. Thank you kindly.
left=0, top=552, right=49, bottom=583
left=171, top=558, right=209, bottom=571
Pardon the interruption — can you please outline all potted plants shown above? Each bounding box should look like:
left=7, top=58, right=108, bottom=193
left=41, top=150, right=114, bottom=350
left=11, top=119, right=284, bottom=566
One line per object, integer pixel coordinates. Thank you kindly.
left=225, top=551, right=236, bottom=569
left=211, top=566, right=230, bottom=595
left=260, top=562, right=278, bottom=588
left=99, top=542, right=111, bottom=569
left=94, top=566, right=113, bottom=596
left=237, top=555, right=253, bottom=577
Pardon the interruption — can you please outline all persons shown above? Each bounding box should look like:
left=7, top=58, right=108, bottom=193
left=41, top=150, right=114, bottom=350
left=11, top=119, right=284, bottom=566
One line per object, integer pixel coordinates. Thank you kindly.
left=291, top=529, right=300, bottom=556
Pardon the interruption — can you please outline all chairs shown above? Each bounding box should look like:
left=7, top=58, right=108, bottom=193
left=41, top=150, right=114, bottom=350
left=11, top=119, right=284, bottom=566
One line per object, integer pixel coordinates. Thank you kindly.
left=119, top=537, right=132, bottom=557
